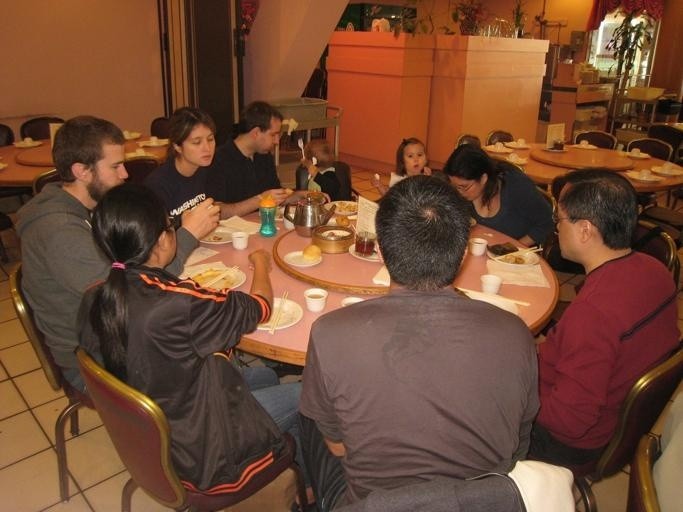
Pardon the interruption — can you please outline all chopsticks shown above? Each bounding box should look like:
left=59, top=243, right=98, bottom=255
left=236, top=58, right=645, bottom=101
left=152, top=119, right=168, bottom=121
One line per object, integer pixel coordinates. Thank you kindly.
left=201, top=265, right=240, bottom=288
left=486, top=245, right=542, bottom=262
left=456, top=286, right=532, bottom=307
left=269, top=290, right=290, bottom=335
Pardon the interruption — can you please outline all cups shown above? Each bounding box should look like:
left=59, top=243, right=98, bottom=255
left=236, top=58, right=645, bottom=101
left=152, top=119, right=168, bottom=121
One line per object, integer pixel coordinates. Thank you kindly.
left=231, top=232, right=250, bottom=251
left=554, top=138, right=563, bottom=150
left=480, top=274, right=502, bottom=294
left=518, top=137, right=525, bottom=146
left=580, top=140, right=589, bottom=148
left=303, top=287, right=328, bottom=313
left=355, top=231, right=377, bottom=257
left=469, top=238, right=487, bottom=258
left=483, top=14, right=517, bottom=38
left=341, top=296, right=363, bottom=306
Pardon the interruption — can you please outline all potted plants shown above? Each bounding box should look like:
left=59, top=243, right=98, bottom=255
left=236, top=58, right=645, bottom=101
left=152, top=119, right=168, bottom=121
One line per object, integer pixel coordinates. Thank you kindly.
left=510, top=0, right=525, bottom=39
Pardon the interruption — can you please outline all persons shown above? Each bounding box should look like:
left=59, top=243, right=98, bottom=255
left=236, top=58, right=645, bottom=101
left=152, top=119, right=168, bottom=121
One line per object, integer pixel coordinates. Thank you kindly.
left=13, top=115, right=220, bottom=398
left=528, top=169, right=683, bottom=466
left=443, top=143, right=553, bottom=248
left=370, top=138, right=432, bottom=197
left=77, top=184, right=317, bottom=512
left=300, top=176, right=541, bottom=512
left=299, top=139, right=341, bottom=202
left=208, top=102, right=331, bottom=220
left=139, top=105, right=216, bottom=231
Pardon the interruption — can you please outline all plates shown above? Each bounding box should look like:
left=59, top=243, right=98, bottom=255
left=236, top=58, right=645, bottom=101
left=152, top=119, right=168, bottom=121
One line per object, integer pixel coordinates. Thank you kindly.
left=190, top=268, right=246, bottom=290
left=481, top=141, right=683, bottom=183
left=463, top=293, right=520, bottom=317
left=199, top=226, right=239, bottom=246
left=0, top=133, right=170, bottom=171
left=282, top=251, right=323, bottom=269
left=348, top=243, right=383, bottom=261
left=324, top=200, right=359, bottom=216
left=486, top=246, right=542, bottom=267
left=256, top=297, right=304, bottom=331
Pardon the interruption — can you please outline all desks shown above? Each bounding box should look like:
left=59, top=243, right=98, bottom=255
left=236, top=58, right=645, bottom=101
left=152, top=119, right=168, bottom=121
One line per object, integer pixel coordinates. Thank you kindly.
left=179, top=201, right=560, bottom=368
left=266, top=96, right=344, bottom=176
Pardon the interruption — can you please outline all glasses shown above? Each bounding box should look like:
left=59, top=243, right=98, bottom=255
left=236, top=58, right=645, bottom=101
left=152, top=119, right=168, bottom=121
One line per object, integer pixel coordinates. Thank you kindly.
left=552, top=199, right=599, bottom=229
left=456, top=172, right=488, bottom=191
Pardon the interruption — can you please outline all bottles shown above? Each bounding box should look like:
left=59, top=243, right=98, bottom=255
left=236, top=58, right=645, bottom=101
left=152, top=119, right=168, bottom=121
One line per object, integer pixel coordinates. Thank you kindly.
left=257, top=194, right=278, bottom=238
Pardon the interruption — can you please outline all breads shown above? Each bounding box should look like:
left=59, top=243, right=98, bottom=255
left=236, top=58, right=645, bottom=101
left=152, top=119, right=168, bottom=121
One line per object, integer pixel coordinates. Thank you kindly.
left=335, top=215, right=350, bottom=227
left=303, top=245, right=321, bottom=261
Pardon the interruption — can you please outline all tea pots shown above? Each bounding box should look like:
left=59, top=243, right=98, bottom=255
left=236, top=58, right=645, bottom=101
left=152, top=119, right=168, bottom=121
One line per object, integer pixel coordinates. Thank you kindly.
left=282, top=192, right=338, bottom=238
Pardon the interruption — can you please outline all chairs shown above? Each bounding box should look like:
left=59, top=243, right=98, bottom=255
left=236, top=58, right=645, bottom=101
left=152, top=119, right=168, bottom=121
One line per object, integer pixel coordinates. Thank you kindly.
left=75, top=347, right=310, bottom=512
left=9, top=261, right=98, bottom=503
left=0, top=117, right=170, bottom=272
left=570, top=345, right=683, bottom=511
left=454, top=82, right=683, bottom=273
